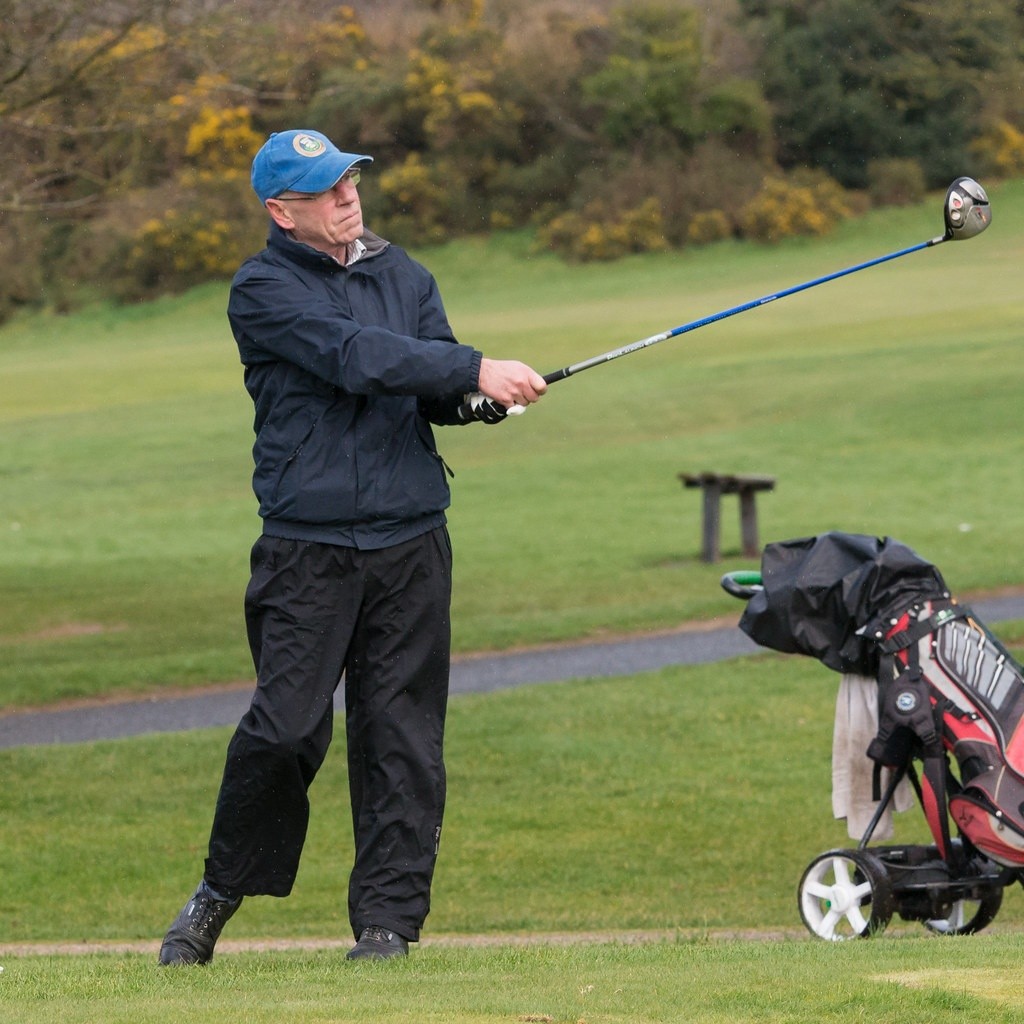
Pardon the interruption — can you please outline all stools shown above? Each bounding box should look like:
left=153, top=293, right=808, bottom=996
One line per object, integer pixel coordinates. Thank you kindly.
left=679, top=469, right=774, bottom=561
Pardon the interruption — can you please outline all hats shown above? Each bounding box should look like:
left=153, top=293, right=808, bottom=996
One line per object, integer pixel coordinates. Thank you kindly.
left=250, top=128, right=374, bottom=208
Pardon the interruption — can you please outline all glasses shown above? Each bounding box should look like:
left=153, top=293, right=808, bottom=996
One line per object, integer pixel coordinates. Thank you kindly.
left=275, top=167, right=362, bottom=201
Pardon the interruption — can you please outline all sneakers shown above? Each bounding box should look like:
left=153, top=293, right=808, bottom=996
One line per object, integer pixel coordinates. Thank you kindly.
left=345, top=925, right=409, bottom=960
left=160, top=879, right=243, bottom=965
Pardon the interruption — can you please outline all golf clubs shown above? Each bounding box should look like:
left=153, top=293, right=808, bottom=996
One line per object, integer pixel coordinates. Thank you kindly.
left=457, top=177, right=993, bottom=420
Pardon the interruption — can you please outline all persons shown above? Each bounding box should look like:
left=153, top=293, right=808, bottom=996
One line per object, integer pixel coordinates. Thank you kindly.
left=160, top=130, right=547, bottom=967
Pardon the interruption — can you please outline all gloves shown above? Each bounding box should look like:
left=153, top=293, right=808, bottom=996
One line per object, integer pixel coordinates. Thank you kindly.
left=461, top=391, right=525, bottom=426
